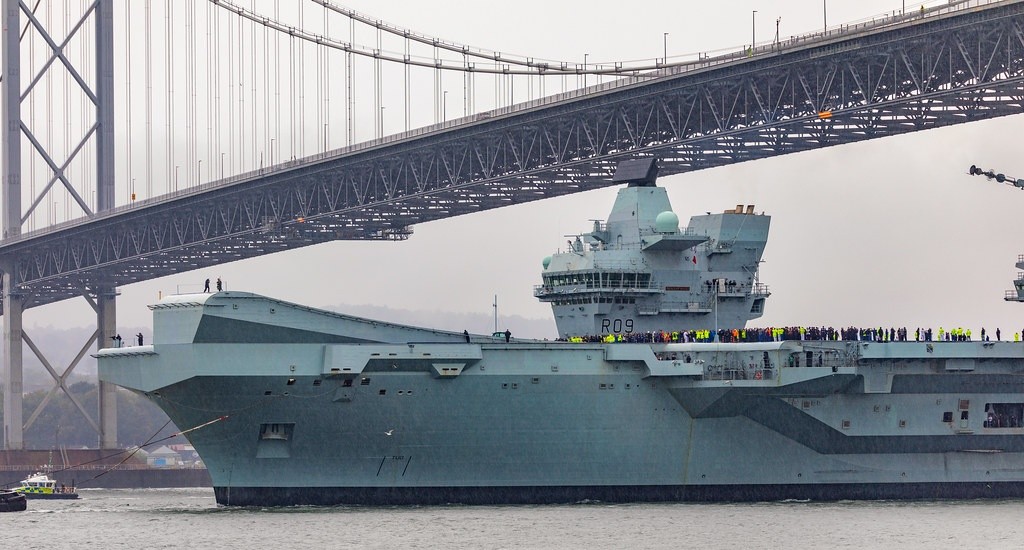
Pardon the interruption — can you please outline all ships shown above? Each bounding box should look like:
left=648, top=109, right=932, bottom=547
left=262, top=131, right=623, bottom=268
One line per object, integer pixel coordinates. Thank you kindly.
left=91, top=158, right=1024, bottom=510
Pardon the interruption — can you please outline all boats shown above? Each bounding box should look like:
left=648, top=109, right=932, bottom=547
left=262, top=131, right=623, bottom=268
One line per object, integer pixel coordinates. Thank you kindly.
left=2, top=445, right=81, bottom=500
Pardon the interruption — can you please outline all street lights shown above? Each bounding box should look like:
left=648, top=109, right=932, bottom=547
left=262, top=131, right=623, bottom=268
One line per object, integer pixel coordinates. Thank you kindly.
left=175, top=165, right=181, bottom=193
left=324, top=123, right=330, bottom=155
left=221, top=152, right=227, bottom=181
left=753, top=10, right=757, bottom=50
left=443, top=90, right=446, bottom=129
left=584, top=53, right=589, bottom=95
left=198, top=159, right=203, bottom=185
left=270, top=137, right=277, bottom=167
left=132, top=178, right=137, bottom=207
left=380, top=107, right=386, bottom=140
left=54, top=201, right=58, bottom=224
left=92, top=190, right=97, bottom=214
left=663, top=33, right=670, bottom=76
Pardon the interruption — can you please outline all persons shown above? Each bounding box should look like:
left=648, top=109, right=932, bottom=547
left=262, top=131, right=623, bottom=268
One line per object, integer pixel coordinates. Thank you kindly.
left=217, top=279, right=222, bottom=291
left=505, top=329, right=511, bottom=342
left=110, top=336, right=116, bottom=340
left=1022, top=329, right=1024, bottom=342
left=204, top=279, right=210, bottom=293
left=555, top=326, right=972, bottom=344
left=136, top=333, right=143, bottom=346
left=981, top=328, right=985, bottom=341
left=996, top=328, right=1001, bottom=341
left=464, top=330, right=470, bottom=344
left=1014, top=333, right=1019, bottom=341
left=116, top=334, right=122, bottom=347
